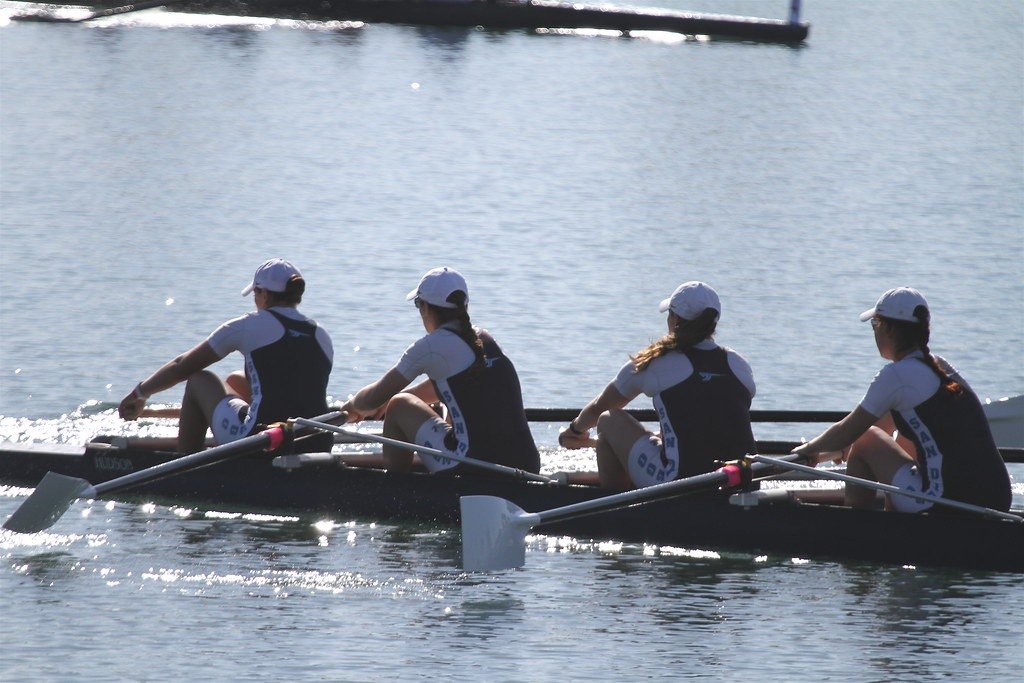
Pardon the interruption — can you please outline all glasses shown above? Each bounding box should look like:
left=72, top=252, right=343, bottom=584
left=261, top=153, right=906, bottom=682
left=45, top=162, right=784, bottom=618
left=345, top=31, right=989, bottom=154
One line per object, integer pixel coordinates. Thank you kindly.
left=870, top=319, right=884, bottom=328
left=414, top=297, right=424, bottom=307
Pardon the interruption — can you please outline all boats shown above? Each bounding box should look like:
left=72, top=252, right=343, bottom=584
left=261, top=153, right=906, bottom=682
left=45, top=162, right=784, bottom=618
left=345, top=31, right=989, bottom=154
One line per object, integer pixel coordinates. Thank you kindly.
left=15, top=0, right=812, bottom=46
left=0, top=420, right=1024, bottom=575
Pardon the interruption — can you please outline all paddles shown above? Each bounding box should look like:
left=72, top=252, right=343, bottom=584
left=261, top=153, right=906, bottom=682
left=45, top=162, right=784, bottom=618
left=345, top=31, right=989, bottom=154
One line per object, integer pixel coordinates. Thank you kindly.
left=1, top=409, right=364, bottom=535
left=118, top=394, right=1024, bottom=449
left=458, top=449, right=845, bottom=571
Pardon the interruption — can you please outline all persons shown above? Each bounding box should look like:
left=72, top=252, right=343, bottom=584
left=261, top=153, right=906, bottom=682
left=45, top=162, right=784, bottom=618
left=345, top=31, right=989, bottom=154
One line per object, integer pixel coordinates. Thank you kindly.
left=791, top=285, right=1012, bottom=514
left=118, top=257, right=334, bottom=452
left=341, top=266, right=540, bottom=477
left=558, top=281, right=760, bottom=491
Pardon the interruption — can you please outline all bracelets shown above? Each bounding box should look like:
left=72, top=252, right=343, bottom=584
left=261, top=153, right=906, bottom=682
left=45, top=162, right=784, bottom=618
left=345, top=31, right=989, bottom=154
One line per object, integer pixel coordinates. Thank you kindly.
left=570, top=417, right=583, bottom=435
left=131, top=381, right=150, bottom=401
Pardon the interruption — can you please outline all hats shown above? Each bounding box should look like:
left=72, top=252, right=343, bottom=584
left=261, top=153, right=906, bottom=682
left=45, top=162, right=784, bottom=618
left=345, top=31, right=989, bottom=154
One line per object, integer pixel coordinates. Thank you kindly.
left=406, top=267, right=468, bottom=308
left=241, top=258, right=302, bottom=297
left=659, top=281, right=721, bottom=322
left=859, top=285, right=931, bottom=323
